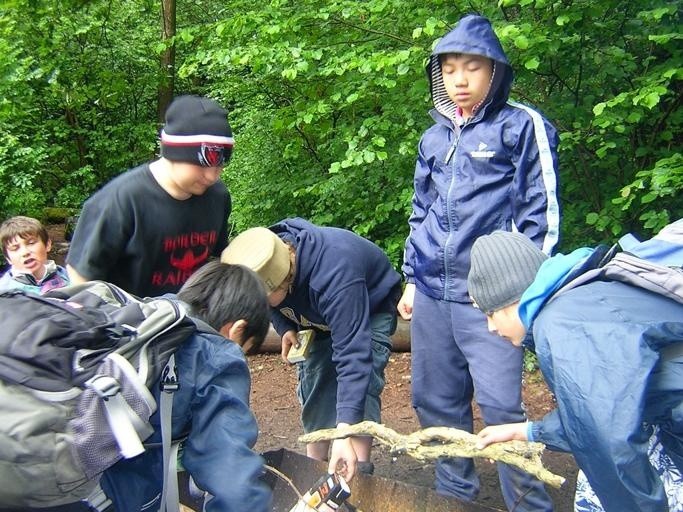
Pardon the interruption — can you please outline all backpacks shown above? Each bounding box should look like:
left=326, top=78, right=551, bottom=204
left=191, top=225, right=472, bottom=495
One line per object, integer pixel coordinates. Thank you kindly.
left=0, top=278, right=227, bottom=512
left=547, top=213, right=683, bottom=311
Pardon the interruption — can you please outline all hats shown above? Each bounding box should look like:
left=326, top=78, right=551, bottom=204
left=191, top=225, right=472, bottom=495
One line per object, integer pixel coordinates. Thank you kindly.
left=466, top=230, right=549, bottom=313
left=160, top=93, right=236, bottom=168
left=220, top=226, right=291, bottom=297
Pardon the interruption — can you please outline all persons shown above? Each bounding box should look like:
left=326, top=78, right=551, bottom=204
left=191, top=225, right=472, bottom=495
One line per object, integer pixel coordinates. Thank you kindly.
left=396, top=16, right=563, bottom=510
left=220, top=216, right=404, bottom=484
left=0, top=215, right=70, bottom=298
left=65, top=93, right=235, bottom=298
left=45, top=260, right=274, bottom=512
left=468, top=227, right=683, bottom=511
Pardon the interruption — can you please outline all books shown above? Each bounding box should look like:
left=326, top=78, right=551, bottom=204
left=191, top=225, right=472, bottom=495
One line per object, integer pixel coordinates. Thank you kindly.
left=290, top=472, right=352, bottom=512
